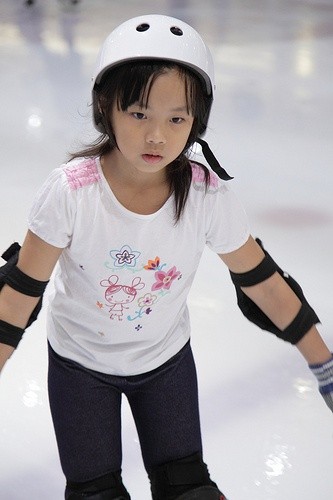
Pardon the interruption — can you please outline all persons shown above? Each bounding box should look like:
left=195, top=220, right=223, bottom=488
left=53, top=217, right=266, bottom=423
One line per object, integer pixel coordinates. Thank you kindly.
left=1, top=15, right=333, bottom=500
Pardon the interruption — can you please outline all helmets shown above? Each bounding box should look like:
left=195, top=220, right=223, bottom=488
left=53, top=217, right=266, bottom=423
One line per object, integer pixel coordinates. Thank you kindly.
left=91, top=15, right=214, bottom=154
